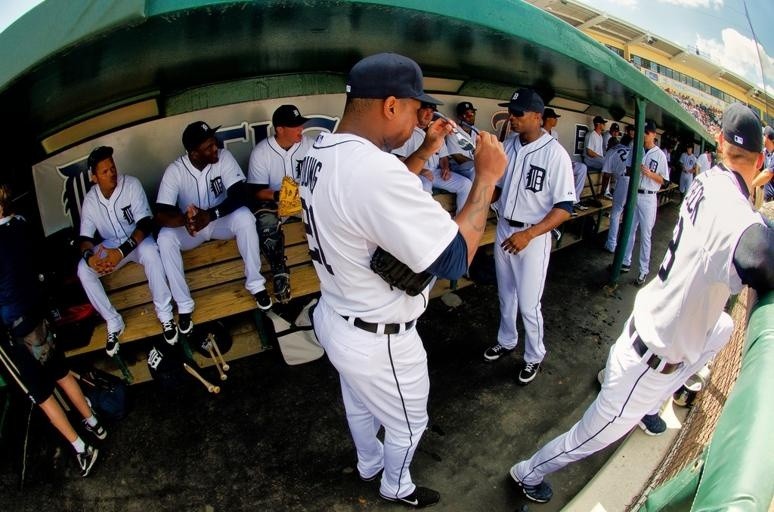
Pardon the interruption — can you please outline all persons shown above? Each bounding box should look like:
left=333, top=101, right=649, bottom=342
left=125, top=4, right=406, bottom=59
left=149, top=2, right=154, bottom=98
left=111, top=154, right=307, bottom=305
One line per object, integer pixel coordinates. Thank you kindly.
left=509, top=103, right=773, bottom=503
left=654, top=87, right=773, bottom=207
left=392, top=102, right=472, bottom=217
left=156, top=122, right=274, bottom=335
left=449, top=101, right=482, bottom=182
left=604, top=122, right=621, bottom=152
left=583, top=116, right=613, bottom=200
left=598, top=136, right=631, bottom=253
left=77, top=146, right=178, bottom=358
left=540, top=108, right=589, bottom=217
left=623, top=125, right=635, bottom=148
left=247, top=103, right=317, bottom=305
left=311, top=50, right=508, bottom=510
left=0, top=195, right=109, bottom=475
left=608, top=118, right=670, bottom=285
left=484, top=86, right=575, bottom=384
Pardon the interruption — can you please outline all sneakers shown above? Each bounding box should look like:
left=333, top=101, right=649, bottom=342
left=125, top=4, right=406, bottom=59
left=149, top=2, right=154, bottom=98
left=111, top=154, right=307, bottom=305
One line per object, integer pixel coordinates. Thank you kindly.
left=571, top=202, right=589, bottom=217
left=160, top=311, right=193, bottom=345
left=378, top=484, right=441, bottom=508
left=83, top=420, right=107, bottom=441
left=76, top=444, right=99, bottom=477
left=603, top=192, right=613, bottom=200
left=252, top=272, right=291, bottom=311
left=638, top=414, right=668, bottom=436
left=505, top=469, right=553, bottom=503
left=105, top=324, right=126, bottom=357
left=619, top=264, right=631, bottom=273
left=361, top=467, right=386, bottom=492
left=517, top=361, right=541, bottom=383
left=636, top=272, right=647, bottom=285
left=484, top=340, right=516, bottom=361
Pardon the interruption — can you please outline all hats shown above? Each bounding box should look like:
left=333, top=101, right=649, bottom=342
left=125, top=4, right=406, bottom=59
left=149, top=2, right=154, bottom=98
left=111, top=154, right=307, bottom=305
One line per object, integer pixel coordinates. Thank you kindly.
left=762, top=125, right=774, bottom=140
left=181, top=121, right=222, bottom=154
left=666, top=139, right=694, bottom=150
left=455, top=102, right=477, bottom=115
left=720, top=100, right=763, bottom=153
left=609, top=120, right=656, bottom=134
left=541, top=107, right=562, bottom=120
left=345, top=53, right=444, bottom=112
left=497, top=88, right=545, bottom=116
left=86, top=145, right=114, bottom=172
left=592, top=116, right=608, bottom=125
left=271, top=105, right=311, bottom=128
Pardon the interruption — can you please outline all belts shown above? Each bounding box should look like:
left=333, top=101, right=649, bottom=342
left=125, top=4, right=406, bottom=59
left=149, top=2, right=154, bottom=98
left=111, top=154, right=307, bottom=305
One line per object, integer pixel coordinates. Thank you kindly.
left=503, top=218, right=537, bottom=228
left=637, top=189, right=657, bottom=194
left=628, top=312, right=685, bottom=375
left=620, top=172, right=631, bottom=176
left=340, top=313, right=417, bottom=336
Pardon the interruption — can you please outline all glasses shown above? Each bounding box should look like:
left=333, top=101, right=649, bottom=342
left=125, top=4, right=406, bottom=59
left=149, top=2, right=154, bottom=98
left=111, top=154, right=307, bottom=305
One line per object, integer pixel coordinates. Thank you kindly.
left=507, top=108, right=534, bottom=118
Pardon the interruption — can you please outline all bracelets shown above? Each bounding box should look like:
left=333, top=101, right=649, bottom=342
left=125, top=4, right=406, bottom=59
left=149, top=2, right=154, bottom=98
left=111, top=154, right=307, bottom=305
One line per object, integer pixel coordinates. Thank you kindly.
left=82, top=248, right=95, bottom=267
left=118, top=237, right=138, bottom=258
left=273, top=190, right=279, bottom=201
left=213, top=206, right=220, bottom=219
